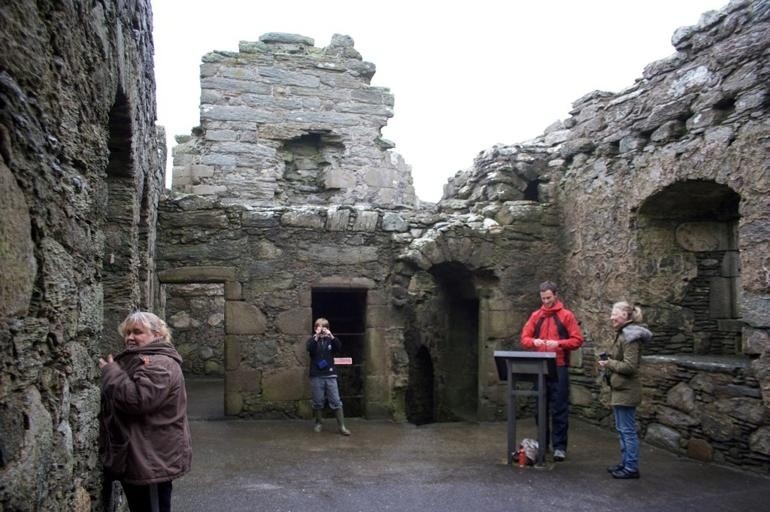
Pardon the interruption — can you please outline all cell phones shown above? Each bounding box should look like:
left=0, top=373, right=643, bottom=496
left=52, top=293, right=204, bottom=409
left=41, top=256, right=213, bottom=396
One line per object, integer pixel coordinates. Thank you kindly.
left=599, top=351, right=608, bottom=360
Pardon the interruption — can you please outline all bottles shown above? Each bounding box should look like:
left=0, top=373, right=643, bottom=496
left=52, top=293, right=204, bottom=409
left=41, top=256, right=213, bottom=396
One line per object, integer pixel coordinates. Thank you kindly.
left=598, top=352, right=607, bottom=361
left=518, top=444, right=527, bottom=466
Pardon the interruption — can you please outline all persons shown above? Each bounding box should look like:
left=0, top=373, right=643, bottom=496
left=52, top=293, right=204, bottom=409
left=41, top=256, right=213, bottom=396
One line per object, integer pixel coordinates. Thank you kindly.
left=521, top=281, right=584, bottom=462
left=306, top=318, right=351, bottom=435
left=99, top=312, right=192, bottom=512
left=598, top=302, right=653, bottom=479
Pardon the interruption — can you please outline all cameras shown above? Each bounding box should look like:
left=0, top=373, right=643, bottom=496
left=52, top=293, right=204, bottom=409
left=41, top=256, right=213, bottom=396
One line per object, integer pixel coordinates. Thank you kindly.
left=320, top=328, right=326, bottom=334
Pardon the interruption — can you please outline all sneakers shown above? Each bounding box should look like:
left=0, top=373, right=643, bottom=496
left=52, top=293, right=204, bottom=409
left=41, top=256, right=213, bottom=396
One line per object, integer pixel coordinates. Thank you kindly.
left=612, top=469, right=640, bottom=479
left=553, top=449, right=566, bottom=461
left=607, top=464, right=624, bottom=474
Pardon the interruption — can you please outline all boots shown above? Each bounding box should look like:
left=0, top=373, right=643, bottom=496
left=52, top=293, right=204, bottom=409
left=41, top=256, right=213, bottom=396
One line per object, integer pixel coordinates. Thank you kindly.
left=314, top=409, right=322, bottom=433
left=336, top=408, right=351, bottom=436
